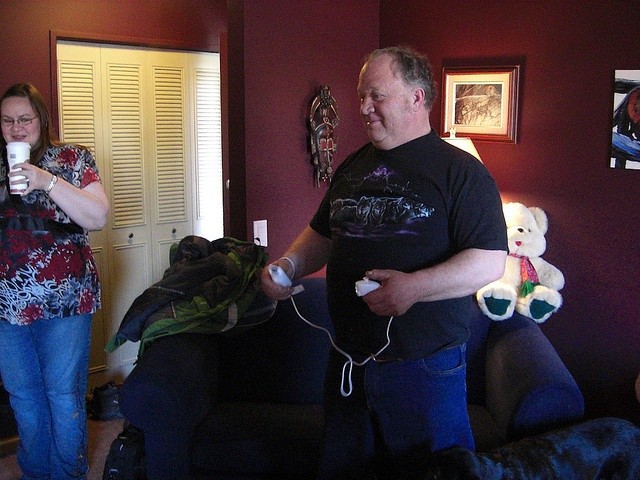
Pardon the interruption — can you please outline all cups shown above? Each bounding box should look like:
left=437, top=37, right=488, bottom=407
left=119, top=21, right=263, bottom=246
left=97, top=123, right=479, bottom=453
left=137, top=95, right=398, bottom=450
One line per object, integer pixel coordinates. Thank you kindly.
left=6, top=141, right=31, bottom=195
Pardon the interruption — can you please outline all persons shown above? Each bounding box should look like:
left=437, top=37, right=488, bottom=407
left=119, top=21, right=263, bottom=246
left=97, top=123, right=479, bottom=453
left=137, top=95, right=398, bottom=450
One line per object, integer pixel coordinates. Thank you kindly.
left=261, top=46, right=509, bottom=470
left=0, top=83, right=110, bottom=479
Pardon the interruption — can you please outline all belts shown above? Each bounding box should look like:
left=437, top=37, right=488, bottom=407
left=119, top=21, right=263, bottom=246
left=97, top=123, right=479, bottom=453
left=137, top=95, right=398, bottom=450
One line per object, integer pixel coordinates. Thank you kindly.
left=360, top=352, right=397, bottom=364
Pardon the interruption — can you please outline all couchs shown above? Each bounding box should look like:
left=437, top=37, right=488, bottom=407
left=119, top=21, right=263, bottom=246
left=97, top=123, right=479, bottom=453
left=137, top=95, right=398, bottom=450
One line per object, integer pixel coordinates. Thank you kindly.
left=116, top=277, right=585, bottom=479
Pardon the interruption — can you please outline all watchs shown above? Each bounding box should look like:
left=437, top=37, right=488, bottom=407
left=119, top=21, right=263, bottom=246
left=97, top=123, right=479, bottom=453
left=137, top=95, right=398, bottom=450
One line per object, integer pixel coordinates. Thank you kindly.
left=45, top=175, right=58, bottom=195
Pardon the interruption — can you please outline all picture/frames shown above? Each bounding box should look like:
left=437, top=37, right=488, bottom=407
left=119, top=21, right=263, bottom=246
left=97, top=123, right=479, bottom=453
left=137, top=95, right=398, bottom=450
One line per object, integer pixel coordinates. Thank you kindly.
left=439, top=63, right=521, bottom=145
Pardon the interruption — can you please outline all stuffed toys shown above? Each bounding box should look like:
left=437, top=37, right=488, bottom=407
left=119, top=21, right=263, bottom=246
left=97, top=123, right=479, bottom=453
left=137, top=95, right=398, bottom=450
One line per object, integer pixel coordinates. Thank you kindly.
left=476, top=202, right=565, bottom=323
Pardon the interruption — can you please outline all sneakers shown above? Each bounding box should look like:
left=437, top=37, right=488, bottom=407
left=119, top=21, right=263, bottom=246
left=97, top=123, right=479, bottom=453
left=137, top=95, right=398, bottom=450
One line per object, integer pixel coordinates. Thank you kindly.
left=94, top=378, right=127, bottom=420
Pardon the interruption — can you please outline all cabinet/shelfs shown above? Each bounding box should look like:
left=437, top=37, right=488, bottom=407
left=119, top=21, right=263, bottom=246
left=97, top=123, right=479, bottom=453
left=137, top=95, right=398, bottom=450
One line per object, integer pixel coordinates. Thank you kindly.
left=56, top=43, right=196, bottom=375
